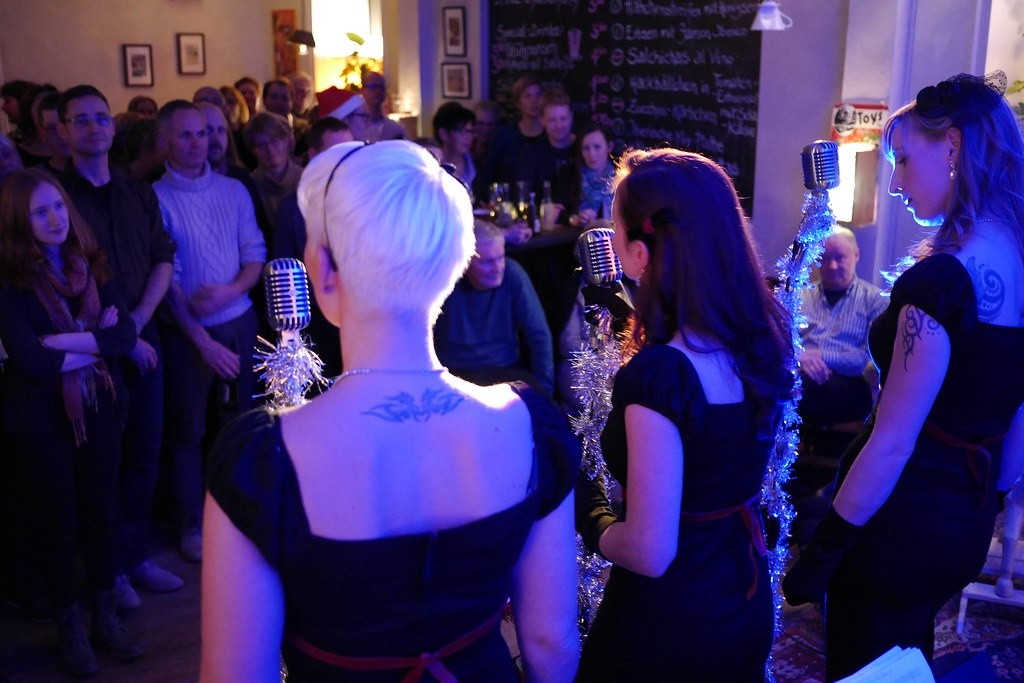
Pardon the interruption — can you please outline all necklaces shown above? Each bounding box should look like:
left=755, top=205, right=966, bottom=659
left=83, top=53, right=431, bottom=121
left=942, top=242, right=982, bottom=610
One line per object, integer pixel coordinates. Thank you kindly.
left=332, top=365, right=450, bottom=387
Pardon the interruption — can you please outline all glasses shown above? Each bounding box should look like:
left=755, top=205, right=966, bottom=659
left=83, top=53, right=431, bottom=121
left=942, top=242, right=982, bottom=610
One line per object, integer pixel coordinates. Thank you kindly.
left=255, top=137, right=284, bottom=150
left=63, top=115, right=113, bottom=129
left=365, top=83, right=384, bottom=89
left=448, top=128, right=478, bottom=138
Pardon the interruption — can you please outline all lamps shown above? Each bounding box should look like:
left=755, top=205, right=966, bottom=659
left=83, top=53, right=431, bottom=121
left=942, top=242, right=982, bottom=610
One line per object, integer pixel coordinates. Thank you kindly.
left=749, top=0, right=793, bottom=32
left=280, top=25, right=316, bottom=56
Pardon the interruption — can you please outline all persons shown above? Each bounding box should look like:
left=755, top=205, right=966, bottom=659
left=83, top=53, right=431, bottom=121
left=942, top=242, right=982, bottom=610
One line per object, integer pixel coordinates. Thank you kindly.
left=782, top=73, right=1024, bottom=683
left=0, top=69, right=888, bottom=683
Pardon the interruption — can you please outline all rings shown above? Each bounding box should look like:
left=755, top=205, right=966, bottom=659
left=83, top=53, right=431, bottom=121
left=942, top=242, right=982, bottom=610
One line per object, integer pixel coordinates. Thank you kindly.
left=810, top=366, right=814, bottom=369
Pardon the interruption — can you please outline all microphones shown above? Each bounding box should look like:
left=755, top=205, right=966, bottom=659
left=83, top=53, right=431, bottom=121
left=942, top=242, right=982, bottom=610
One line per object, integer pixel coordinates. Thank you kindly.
left=576, top=227, right=623, bottom=288
left=801, top=139, right=841, bottom=198
left=264, top=258, right=311, bottom=347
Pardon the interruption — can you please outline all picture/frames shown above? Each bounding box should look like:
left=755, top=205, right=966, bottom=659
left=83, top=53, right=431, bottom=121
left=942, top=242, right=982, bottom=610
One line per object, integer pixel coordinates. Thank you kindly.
left=442, top=6, right=467, bottom=58
left=441, top=62, right=473, bottom=99
left=177, top=32, right=206, bottom=76
left=122, top=43, right=154, bottom=88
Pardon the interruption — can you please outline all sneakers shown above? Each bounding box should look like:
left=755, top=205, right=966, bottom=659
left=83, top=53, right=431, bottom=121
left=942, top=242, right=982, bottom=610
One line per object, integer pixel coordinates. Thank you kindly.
left=58, top=624, right=100, bottom=678
left=90, top=608, right=145, bottom=660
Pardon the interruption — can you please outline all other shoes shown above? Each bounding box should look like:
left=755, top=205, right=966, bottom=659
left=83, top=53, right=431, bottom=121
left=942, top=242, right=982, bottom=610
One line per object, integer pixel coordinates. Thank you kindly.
left=107, top=574, right=140, bottom=609
left=180, top=526, right=201, bottom=562
left=130, top=560, right=185, bottom=593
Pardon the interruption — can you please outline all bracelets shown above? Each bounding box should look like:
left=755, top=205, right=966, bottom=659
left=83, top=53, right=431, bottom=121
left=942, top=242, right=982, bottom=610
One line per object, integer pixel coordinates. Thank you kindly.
left=39, top=333, right=53, bottom=346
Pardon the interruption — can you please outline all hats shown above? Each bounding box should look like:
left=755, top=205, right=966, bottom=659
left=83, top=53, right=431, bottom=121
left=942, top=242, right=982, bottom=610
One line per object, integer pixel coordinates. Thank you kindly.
left=432, top=101, right=476, bottom=127
